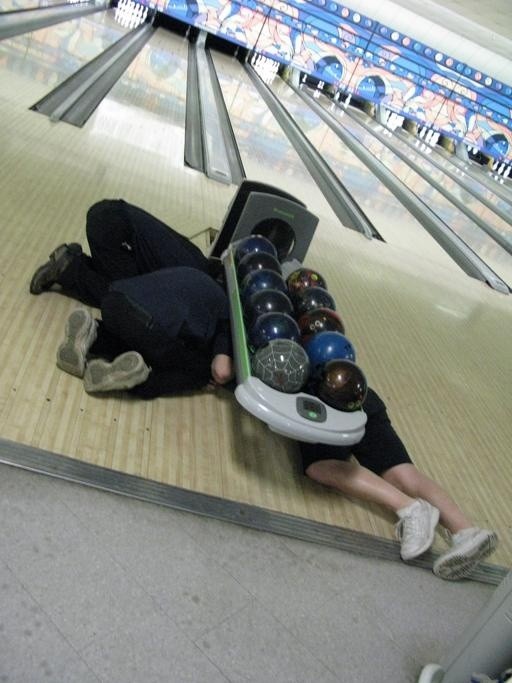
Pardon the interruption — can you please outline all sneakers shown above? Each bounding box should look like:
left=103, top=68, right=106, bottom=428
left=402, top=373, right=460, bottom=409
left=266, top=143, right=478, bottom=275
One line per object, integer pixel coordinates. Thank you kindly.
left=83, top=350, right=150, bottom=395
left=29, top=243, right=84, bottom=293
left=57, top=309, right=98, bottom=378
left=433, top=527, right=499, bottom=580
left=395, top=498, right=440, bottom=560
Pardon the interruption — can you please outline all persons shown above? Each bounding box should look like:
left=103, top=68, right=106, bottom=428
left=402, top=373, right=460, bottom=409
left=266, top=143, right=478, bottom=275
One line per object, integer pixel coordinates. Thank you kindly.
left=29, top=199, right=212, bottom=310
left=55, top=266, right=233, bottom=398
left=301, top=387, right=499, bottom=581
